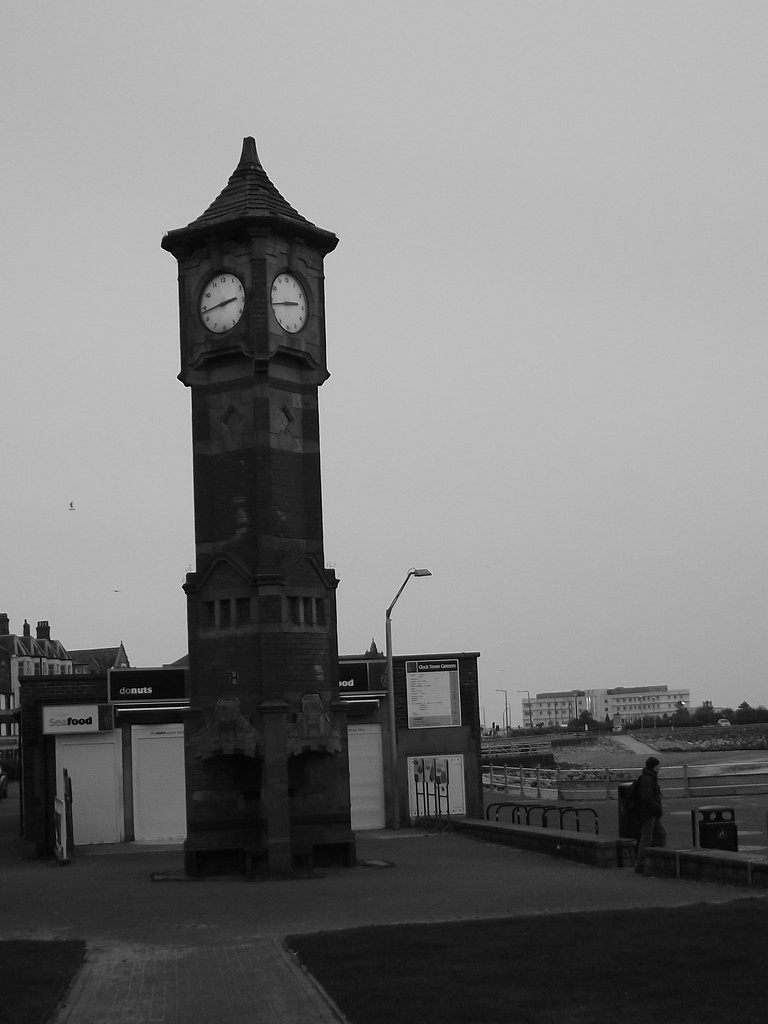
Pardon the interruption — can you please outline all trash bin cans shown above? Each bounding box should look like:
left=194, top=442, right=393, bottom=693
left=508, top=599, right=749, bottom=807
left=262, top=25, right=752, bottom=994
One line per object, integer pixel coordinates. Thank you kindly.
left=691, top=805, right=738, bottom=854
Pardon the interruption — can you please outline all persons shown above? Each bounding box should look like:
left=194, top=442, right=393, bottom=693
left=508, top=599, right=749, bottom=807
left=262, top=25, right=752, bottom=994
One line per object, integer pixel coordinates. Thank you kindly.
left=634, top=757, right=666, bottom=875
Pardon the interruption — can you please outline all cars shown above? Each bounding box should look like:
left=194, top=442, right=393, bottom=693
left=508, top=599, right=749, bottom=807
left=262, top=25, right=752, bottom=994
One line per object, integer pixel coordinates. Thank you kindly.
left=718, top=718, right=730, bottom=727
left=612, top=725, right=623, bottom=733
left=0, top=767, right=9, bottom=799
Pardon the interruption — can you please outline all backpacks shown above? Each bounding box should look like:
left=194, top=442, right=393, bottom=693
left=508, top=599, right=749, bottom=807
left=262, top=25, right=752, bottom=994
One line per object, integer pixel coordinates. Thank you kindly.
left=624, top=779, right=640, bottom=813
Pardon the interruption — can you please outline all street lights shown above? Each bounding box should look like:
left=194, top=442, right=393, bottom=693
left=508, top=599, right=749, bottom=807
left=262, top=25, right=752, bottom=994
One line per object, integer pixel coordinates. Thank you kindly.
left=651, top=696, right=657, bottom=729
left=638, top=695, right=644, bottom=729
left=496, top=689, right=508, bottom=737
left=545, top=695, right=558, bottom=728
left=518, top=690, right=533, bottom=728
left=386, top=567, right=433, bottom=834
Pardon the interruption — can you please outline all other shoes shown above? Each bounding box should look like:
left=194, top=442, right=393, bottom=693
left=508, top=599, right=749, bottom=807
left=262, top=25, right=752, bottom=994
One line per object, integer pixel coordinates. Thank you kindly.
left=635, top=863, right=652, bottom=876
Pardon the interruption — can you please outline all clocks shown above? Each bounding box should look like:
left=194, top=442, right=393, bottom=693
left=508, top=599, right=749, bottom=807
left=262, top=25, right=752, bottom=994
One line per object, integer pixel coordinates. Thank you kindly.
left=271, top=272, right=309, bottom=333
left=198, top=271, right=246, bottom=334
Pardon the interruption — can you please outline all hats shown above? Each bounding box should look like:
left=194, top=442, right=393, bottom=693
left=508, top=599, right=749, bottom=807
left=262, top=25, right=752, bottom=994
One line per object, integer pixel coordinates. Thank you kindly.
left=646, top=757, right=659, bottom=770
